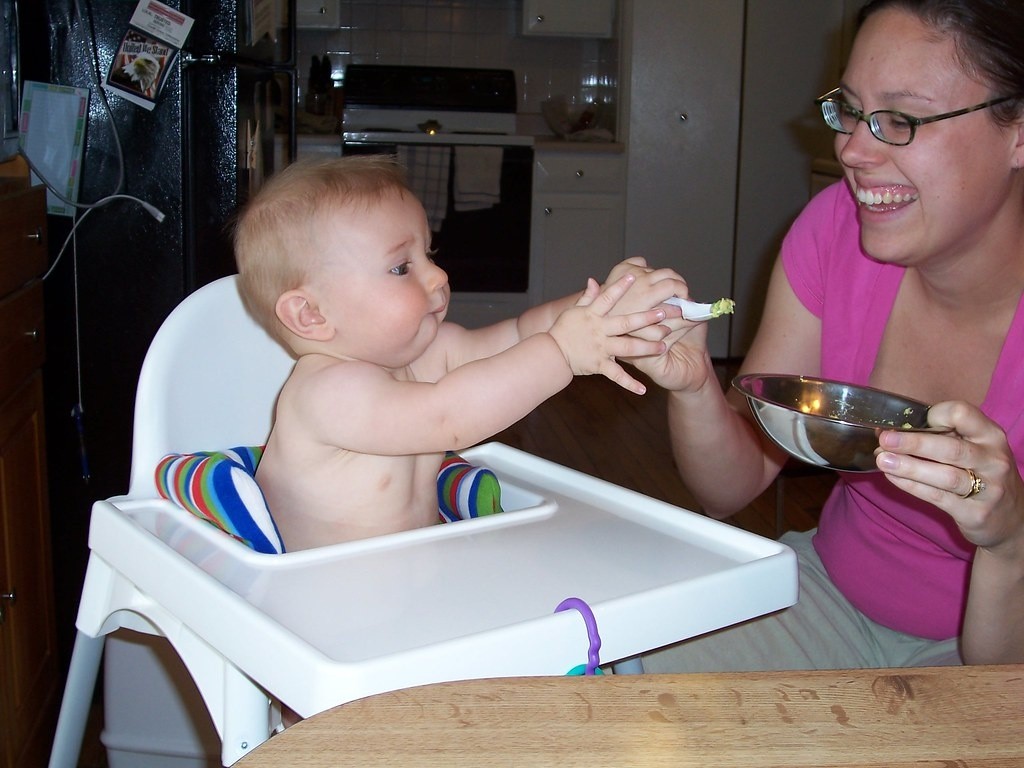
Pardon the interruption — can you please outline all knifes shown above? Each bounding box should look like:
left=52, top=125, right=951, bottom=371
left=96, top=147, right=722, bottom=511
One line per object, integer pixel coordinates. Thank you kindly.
left=308, top=54, right=336, bottom=96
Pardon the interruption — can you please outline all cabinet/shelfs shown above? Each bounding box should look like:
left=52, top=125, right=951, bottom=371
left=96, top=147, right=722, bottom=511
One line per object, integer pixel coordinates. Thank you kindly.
left=273, top=0, right=841, bottom=359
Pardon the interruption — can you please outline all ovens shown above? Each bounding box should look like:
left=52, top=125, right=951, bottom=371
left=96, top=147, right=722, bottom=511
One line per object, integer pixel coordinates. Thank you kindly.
left=342, top=142, right=534, bottom=319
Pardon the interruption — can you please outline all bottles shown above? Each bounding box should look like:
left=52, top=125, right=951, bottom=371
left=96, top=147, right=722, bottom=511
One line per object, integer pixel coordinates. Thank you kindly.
left=571, top=97, right=603, bottom=133
left=308, top=94, right=323, bottom=116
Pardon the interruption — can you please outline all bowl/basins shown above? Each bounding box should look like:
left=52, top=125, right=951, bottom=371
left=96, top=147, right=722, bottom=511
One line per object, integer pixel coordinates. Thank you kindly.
left=731, top=373, right=957, bottom=475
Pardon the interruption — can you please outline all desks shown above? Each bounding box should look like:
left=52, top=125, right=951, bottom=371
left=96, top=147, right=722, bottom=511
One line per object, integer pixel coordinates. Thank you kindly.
left=230, top=662, right=1024, bottom=768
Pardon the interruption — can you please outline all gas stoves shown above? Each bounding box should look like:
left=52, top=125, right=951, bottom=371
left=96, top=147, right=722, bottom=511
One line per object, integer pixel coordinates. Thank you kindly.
left=343, top=63, right=537, bottom=146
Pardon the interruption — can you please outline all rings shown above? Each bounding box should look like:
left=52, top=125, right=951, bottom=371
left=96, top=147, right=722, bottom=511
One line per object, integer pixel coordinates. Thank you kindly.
left=960, top=469, right=987, bottom=499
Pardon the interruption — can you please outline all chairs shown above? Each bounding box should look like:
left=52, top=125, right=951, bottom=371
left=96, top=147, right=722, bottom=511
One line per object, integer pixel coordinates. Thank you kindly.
left=48, top=274, right=799, bottom=768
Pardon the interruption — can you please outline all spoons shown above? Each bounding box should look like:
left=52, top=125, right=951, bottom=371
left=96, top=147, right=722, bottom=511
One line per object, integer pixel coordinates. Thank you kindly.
left=663, top=297, right=724, bottom=322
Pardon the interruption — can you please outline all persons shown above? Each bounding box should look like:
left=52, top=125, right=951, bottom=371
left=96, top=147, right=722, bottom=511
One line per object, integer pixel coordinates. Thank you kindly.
left=596, top=1, right=1024, bottom=676
left=234, top=152, right=668, bottom=740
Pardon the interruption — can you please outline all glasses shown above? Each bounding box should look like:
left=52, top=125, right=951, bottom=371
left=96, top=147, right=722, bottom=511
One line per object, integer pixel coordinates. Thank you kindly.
left=813, top=88, right=1024, bottom=147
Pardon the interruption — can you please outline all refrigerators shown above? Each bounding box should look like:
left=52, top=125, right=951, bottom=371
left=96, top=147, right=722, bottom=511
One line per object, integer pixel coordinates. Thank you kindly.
left=0, top=0, right=296, bottom=698
